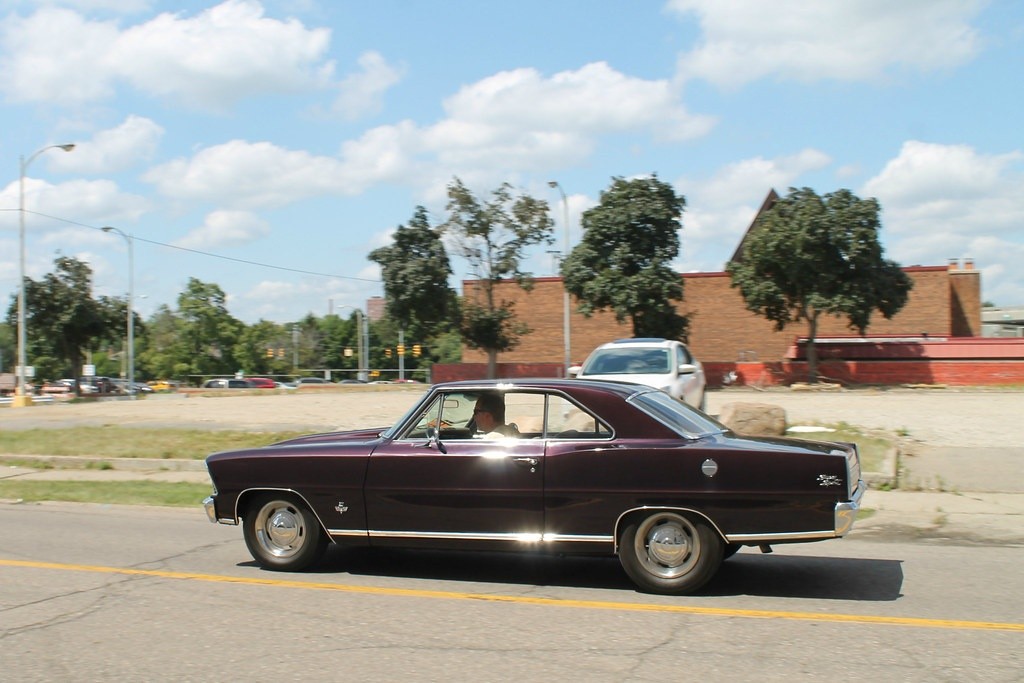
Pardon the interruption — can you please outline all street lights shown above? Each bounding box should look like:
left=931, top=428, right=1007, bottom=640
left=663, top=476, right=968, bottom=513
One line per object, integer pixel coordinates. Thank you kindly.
left=548, top=181, right=570, bottom=377
left=337, top=304, right=368, bottom=371
left=101, top=226, right=134, bottom=385
left=18, top=143, right=75, bottom=397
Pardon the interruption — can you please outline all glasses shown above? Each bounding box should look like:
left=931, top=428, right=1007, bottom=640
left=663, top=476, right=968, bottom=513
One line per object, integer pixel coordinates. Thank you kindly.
left=474, top=409, right=490, bottom=415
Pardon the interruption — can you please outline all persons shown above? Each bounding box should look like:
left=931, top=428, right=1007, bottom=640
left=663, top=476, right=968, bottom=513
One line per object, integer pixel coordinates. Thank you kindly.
left=474, top=395, right=521, bottom=440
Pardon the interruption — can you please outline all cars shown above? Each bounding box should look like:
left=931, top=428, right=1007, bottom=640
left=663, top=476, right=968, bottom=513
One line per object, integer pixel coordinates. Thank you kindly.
left=567, top=338, right=706, bottom=411
left=203, top=378, right=865, bottom=596
left=200, top=378, right=420, bottom=388
left=80, top=376, right=175, bottom=396
